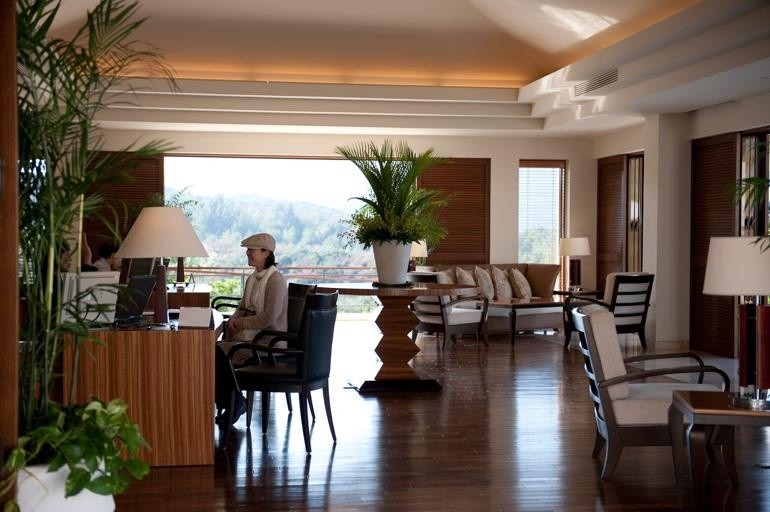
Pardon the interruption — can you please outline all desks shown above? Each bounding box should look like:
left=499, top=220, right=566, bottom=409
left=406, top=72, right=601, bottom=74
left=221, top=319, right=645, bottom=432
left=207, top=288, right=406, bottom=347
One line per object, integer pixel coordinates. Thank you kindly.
left=664, top=388, right=770, bottom=512
left=550, top=289, right=603, bottom=298
left=62, top=306, right=219, bottom=467
left=315, top=280, right=481, bottom=395
left=164, top=278, right=211, bottom=309
left=476, top=298, right=591, bottom=348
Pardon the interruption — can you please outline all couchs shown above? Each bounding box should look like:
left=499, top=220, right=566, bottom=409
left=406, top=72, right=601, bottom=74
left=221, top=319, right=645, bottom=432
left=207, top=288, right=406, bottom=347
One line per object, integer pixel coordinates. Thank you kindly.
left=433, top=260, right=561, bottom=303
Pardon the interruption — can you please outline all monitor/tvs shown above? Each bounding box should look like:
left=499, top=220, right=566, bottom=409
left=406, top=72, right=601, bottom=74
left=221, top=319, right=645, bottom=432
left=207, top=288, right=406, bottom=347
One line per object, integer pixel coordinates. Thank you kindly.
left=122, top=275, right=157, bottom=317
left=126, top=258, right=156, bottom=284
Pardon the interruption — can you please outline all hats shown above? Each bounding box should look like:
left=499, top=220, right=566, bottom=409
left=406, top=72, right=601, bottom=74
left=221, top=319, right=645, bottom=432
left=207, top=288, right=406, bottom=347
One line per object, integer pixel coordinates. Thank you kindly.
left=239, top=233, right=276, bottom=252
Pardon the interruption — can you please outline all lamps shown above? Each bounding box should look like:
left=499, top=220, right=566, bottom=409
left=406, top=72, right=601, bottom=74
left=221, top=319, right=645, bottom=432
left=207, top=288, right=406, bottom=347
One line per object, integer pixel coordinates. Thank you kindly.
left=118, top=205, right=207, bottom=324
left=408, top=238, right=428, bottom=272
left=703, top=236, right=770, bottom=409
left=558, top=237, right=591, bottom=291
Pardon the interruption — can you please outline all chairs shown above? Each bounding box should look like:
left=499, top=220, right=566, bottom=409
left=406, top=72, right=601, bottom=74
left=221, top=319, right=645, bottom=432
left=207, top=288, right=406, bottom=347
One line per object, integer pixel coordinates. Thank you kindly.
left=568, top=304, right=740, bottom=486
left=562, top=268, right=654, bottom=350
left=221, top=279, right=318, bottom=425
left=404, top=271, right=492, bottom=350
left=231, top=290, right=339, bottom=452
left=211, top=295, right=243, bottom=311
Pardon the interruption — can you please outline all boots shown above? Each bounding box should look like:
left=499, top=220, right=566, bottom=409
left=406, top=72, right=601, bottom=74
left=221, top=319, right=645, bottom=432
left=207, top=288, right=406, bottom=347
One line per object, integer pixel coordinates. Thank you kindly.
left=215, top=397, right=248, bottom=427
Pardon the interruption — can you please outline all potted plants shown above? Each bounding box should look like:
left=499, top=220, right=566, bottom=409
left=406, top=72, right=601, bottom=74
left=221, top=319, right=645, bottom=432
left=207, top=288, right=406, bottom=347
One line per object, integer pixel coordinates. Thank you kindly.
left=0, top=395, right=157, bottom=512
left=333, top=133, right=450, bottom=286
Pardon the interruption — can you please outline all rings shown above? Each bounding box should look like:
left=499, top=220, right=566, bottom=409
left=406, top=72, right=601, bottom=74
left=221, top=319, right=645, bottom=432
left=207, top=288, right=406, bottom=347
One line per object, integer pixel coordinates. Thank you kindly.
left=227, top=324, right=232, bottom=328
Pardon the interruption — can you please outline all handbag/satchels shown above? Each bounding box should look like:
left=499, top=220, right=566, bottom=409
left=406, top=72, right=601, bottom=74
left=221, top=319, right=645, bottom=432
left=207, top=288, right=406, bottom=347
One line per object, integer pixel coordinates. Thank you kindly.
left=232, top=316, right=260, bottom=341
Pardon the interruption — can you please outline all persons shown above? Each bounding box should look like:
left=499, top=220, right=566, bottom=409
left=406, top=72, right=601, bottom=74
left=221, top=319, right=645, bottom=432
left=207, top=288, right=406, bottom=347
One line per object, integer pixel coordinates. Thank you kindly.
left=35, top=232, right=97, bottom=400
left=213, top=233, right=288, bottom=430
left=94, top=242, right=123, bottom=272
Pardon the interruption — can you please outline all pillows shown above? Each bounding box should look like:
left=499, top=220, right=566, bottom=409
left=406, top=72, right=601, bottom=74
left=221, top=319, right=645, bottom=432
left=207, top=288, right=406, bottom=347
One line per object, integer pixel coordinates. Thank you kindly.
left=440, top=268, right=457, bottom=300
left=508, top=266, right=532, bottom=299
left=455, top=267, right=478, bottom=298
left=490, top=265, right=513, bottom=300
left=475, top=265, right=494, bottom=301
